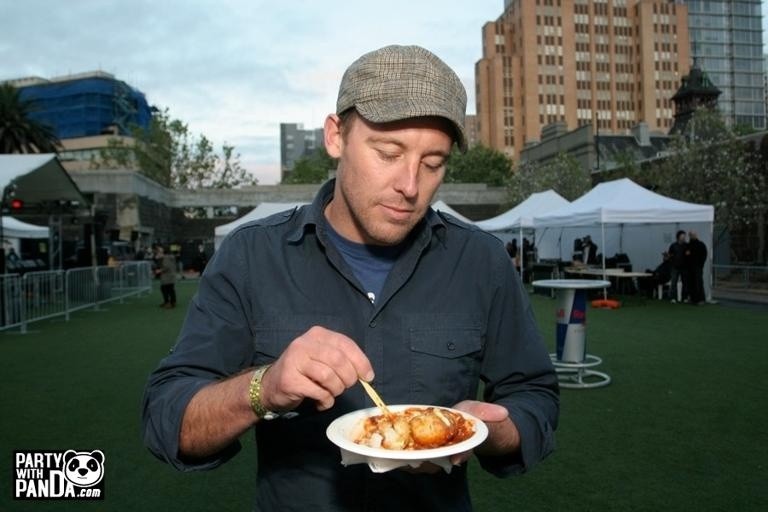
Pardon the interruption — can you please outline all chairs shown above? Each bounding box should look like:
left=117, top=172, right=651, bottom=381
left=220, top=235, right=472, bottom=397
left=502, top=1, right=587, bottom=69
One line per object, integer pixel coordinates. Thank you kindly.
left=653, top=271, right=682, bottom=303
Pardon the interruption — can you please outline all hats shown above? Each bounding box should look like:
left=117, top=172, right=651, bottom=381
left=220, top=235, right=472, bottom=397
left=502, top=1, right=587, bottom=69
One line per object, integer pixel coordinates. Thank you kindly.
left=335, top=42, right=469, bottom=156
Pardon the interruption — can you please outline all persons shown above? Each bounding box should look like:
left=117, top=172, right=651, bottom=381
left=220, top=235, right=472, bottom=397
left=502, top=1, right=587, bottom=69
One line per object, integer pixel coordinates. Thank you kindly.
left=504, top=230, right=708, bottom=308
left=4, top=235, right=213, bottom=310
left=140, top=41, right=561, bottom=510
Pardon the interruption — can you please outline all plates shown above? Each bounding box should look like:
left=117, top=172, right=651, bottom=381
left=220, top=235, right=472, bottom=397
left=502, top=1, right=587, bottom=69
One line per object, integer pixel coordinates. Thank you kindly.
left=325, top=404, right=489, bottom=460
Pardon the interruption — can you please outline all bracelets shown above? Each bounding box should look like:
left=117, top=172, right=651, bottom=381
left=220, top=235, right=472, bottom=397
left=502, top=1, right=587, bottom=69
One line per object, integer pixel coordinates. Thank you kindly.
left=249, top=361, right=291, bottom=422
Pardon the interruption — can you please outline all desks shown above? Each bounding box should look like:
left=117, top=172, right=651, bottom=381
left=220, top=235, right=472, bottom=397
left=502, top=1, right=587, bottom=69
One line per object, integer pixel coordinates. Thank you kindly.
left=529, top=262, right=657, bottom=299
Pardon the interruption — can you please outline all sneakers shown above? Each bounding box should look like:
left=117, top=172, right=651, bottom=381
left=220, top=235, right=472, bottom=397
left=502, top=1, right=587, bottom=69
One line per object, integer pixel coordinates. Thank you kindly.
left=671, top=298, right=704, bottom=305
left=159, top=302, right=176, bottom=309
